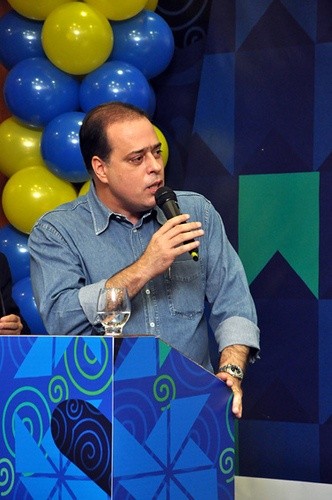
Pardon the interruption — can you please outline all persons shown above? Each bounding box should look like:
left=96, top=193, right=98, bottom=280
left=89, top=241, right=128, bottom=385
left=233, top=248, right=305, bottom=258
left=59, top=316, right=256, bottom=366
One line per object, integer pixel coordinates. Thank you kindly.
left=27, top=103, right=260, bottom=419
left=0, top=252, right=25, bottom=335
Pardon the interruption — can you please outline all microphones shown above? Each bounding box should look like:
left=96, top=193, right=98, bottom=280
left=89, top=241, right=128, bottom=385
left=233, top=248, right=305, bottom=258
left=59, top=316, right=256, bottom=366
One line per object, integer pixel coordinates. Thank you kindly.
left=155, top=186, right=199, bottom=262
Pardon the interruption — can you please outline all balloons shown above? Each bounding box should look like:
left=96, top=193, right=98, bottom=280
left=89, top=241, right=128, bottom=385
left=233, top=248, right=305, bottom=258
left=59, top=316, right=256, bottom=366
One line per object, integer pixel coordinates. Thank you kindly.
left=40, top=111, right=91, bottom=182
left=9, top=1, right=74, bottom=20
left=1, top=114, right=44, bottom=177
left=78, top=178, right=90, bottom=195
left=4, top=57, right=80, bottom=129
left=0, top=11, right=48, bottom=71
left=145, top=0, right=158, bottom=11
left=12, top=277, right=49, bottom=335
left=2, top=166, right=78, bottom=235
left=79, top=60, right=156, bottom=121
left=41, top=2, right=114, bottom=75
left=105, top=9, right=174, bottom=80
left=84, top=0, right=148, bottom=20
left=150, top=123, right=168, bottom=169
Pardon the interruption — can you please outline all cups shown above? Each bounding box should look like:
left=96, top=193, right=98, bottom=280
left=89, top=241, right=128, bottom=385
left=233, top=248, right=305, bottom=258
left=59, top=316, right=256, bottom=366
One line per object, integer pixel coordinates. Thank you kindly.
left=97, top=287, right=132, bottom=336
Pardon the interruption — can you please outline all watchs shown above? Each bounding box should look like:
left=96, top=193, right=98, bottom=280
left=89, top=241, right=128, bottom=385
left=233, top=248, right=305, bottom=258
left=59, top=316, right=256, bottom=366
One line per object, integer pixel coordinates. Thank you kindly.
left=217, top=364, right=245, bottom=380
